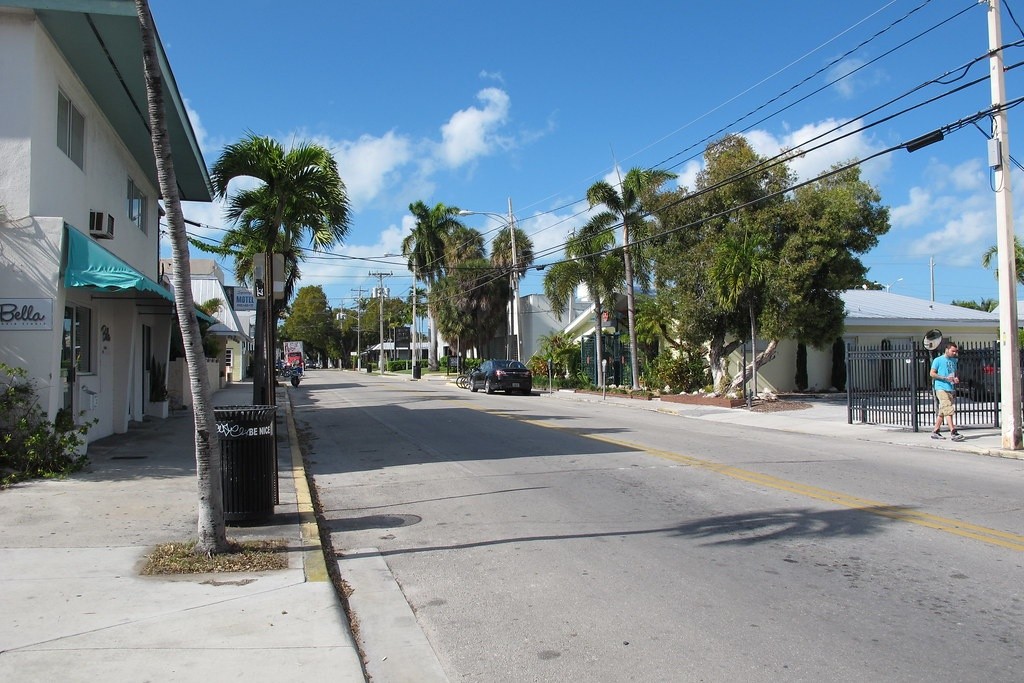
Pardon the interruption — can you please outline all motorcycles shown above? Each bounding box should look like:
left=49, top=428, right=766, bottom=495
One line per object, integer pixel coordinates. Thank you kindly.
left=276, top=359, right=304, bottom=387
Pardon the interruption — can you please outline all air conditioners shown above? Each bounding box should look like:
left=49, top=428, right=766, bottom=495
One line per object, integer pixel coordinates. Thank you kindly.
left=91, top=209, right=115, bottom=240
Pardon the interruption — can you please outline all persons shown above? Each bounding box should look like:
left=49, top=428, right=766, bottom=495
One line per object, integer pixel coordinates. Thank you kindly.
left=930, top=341, right=965, bottom=441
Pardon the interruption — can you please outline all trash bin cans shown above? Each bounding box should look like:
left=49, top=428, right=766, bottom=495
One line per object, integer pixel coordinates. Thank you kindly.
left=213, top=405, right=279, bottom=527
left=367, top=364, right=372, bottom=372
left=413, top=365, right=421, bottom=379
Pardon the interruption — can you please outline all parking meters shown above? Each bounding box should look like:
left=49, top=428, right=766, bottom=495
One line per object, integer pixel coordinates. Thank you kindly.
left=547, top=359, right=553, bottom=394
left=601, top=359, right=608, bottom=400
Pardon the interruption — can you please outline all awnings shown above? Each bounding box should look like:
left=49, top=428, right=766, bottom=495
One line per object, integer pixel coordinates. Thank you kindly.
left=63, top=221, right=215, bottom=327
left=169, top=276, right=252, bottom=343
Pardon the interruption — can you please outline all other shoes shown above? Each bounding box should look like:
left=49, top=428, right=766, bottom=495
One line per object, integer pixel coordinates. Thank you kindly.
left=951, top=433, right=964, bottom=441
left=931, top=432, right=946, bottom=439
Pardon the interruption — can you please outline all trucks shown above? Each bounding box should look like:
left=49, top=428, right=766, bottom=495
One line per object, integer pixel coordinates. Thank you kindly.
left=283, top=340, right=307, bottom=370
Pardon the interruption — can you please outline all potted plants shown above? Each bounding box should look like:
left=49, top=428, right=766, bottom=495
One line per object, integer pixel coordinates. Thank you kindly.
left=198, top=298, right=227, bottom=317
left=219, top=371, right=226, bottom=389
left=148, top=353, right=170, bottom=419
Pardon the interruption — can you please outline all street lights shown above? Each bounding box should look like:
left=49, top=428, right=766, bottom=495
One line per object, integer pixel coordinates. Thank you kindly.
left=383, top=251, right=418, bottom=378
left=459, top=196, right=523, bottom=364
left=342, top=313, right=361, bottom=371
left=873, top=278, right=904, bottom=293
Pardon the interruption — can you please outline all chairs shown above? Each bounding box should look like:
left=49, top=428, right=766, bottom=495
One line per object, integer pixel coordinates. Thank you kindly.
left=511, top=364, right=518, bottom=368
left=495, top=362, right=502, bottom=368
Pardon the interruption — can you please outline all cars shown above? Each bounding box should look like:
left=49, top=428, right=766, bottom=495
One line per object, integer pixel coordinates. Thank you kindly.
left=305, top=358, right=317, bottom=369
left=467, top=359, right=533, bottom=395
left=955, top=350, right=1024, bottom=402
